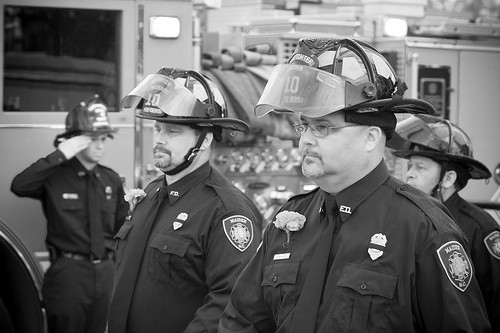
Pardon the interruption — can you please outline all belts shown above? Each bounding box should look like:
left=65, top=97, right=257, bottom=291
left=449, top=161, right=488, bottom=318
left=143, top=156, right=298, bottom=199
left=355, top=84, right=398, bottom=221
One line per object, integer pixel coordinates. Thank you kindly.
left=58, top=250, right=102, bottom=264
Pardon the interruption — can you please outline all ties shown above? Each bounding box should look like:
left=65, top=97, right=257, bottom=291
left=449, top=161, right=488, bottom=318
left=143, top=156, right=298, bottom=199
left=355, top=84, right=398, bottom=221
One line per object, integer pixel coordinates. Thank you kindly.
left=287, top=196, right=336, bottom=333
left=86, top=172, right=107, bottom=260
left=108, top=184, right=168, bottom=333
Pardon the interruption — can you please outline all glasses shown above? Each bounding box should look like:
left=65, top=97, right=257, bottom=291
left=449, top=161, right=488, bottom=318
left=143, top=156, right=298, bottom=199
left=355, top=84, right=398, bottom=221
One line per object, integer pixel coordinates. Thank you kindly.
left=293, top=122, right=358, bottom=138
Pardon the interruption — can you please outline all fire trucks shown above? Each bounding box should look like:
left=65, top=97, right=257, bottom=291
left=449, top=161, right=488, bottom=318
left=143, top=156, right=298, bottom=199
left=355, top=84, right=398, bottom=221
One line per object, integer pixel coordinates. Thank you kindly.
left=1, top=1, right=500, bottom=333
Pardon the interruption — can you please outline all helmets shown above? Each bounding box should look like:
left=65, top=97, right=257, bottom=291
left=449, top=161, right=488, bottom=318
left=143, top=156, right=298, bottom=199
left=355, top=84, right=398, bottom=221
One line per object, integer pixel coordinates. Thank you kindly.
left=134, top=67, right=249, bottom=133
left=385, top=115, right=492, bottom=180
left=57, top=102, right=116, bottom=138
left=274, top=37, right=436, bottom=115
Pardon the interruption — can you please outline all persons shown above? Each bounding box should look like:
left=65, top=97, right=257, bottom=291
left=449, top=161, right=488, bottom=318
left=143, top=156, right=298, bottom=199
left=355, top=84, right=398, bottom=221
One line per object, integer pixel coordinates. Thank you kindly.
left=219, top=37, right=492, bottom=333
left=9, top=99, right=130, bottom=333
left=106, top=70, right=261, bottom=333
left=386, top=114, right=500, bottom=333
